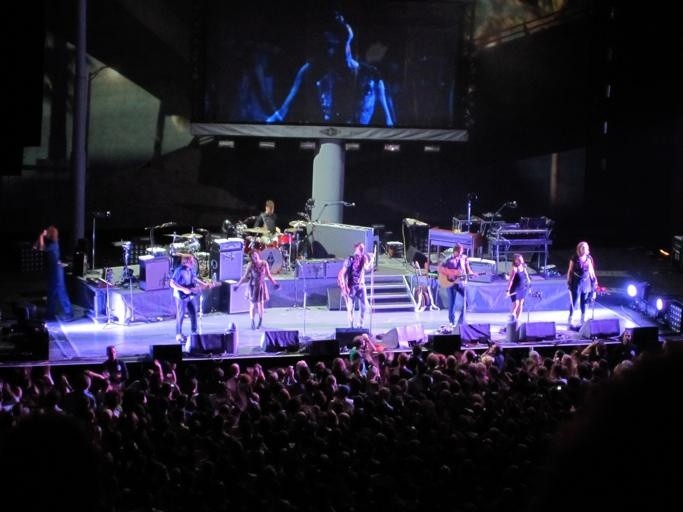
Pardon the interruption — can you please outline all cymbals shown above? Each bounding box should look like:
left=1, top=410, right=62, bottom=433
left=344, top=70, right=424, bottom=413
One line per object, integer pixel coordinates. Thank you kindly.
left=113, top=240, right=131, bottom=246
left=243, top=227, right=270, bottom=234
left=164, top=232, right=202, bottom=239
left=285, top=220, right=307, bottom=232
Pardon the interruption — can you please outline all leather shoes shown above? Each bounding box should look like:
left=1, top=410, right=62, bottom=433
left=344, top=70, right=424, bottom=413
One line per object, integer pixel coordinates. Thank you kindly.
left=257, top=322, right=261, bottom=329
left=251, top=321, right=256, bottom=330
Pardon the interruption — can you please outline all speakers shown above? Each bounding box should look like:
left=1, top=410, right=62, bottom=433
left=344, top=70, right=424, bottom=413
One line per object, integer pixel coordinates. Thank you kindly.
left=209, top=251, right=241, bottom=281
left=140, top=255, right=170, bottom=291
left=262, top=331, right=299, bottom=352
left=579, top=319, right=620, bottom=339
left=407, top=245, right=433, bottom=269
left=517, top=322, right=556, bottom=342
left=184, top=334, right=224, bottom=353
left=382, top=322, right=424, bottom=349
left=336, top=328, right=369, bottom=349
left=451, top=324, right=491, bottom=344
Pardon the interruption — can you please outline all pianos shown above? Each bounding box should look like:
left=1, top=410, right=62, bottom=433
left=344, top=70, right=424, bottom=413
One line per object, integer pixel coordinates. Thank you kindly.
left=430, top=228, right=481, bottom=249
left=489, top=224, right=553, bottom=246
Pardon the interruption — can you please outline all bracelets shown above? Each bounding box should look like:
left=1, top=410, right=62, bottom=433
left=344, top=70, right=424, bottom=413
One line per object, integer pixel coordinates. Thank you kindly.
left=507, top=290, right=510, bottom=292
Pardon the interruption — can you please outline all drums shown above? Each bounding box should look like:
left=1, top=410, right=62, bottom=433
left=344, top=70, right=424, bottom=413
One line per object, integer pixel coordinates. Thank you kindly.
left=244, top=236, right=265, bottom=254
left=277, top=234, right=293, bottom=247
left=259, top=246, right=283, bottom=274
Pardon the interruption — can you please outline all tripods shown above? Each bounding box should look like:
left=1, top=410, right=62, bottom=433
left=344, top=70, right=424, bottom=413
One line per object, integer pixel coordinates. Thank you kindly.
left=280, top=233, right=295, bottom=271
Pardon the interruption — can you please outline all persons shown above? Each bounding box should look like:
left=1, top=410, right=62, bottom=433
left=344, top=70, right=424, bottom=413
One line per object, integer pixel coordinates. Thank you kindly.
left=507, top=253, right=531, bottom=331
left=169, top=255, right=200, bottom=344
left=567, top=241, right=598, bottom=331
left=253, top=200, right=278, bottom=234
left=233, top=250, right=280, bottom=330
left=266, top=10, right=399, bottom=127
left=1, top=334, right=682, bottom=512
left=32, top=225, right=85, bottom=323
left=438, top=242, right=478, bottom=327
left=406, top=244, right=440, bottom=313
left=337, top=242, right=375, bottom=328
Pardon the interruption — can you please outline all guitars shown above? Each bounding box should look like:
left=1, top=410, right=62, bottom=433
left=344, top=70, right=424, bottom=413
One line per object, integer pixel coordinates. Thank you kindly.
left=438, top=268, right=486, bottom=288
left=179, top=282, right=222, bottom=300
